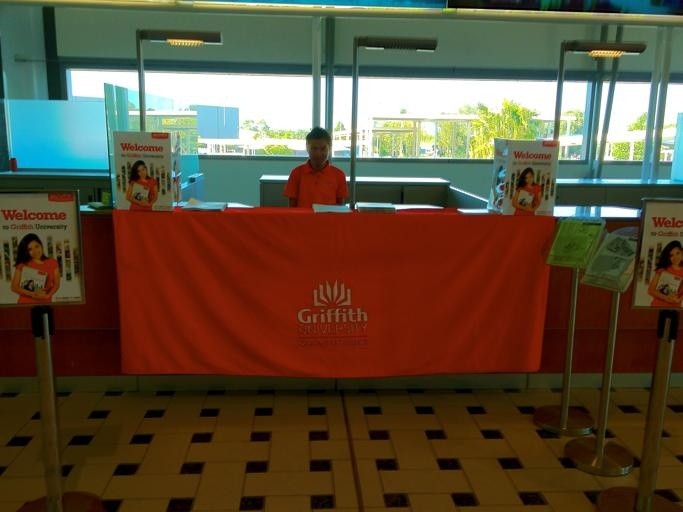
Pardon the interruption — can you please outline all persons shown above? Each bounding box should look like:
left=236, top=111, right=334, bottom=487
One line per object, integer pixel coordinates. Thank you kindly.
left=648, top=240, right=683, bottom=306
left=282, top=127, right=349, bottom=207
left=11, top=233, right=60, bottom=304
left=125, top=160, right=158, bottom=210
left=512, top=167, right=542, bottom=215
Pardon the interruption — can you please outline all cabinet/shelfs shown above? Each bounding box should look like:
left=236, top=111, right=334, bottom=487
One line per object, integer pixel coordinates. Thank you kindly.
left=554, top=176, right=682, bottom=210
left=258, top=176, right=449, bottom=212
